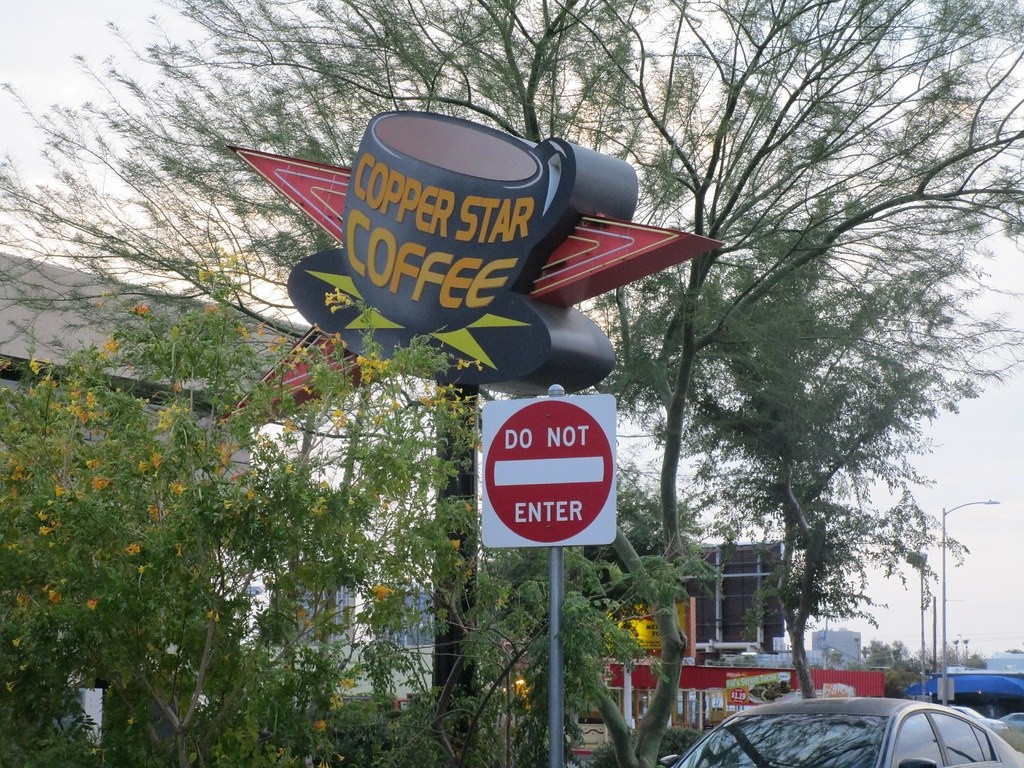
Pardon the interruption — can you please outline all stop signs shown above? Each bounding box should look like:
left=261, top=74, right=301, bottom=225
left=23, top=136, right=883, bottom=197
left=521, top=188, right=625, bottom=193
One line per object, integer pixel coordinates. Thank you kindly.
left=482, top=393, right=616, bottom=546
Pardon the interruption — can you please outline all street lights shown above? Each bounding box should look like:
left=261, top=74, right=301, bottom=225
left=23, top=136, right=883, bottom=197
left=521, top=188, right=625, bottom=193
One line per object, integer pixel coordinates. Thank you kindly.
left=218, top=110, right=725, bottom=768
left=942, top=499, right=1002, bottom=705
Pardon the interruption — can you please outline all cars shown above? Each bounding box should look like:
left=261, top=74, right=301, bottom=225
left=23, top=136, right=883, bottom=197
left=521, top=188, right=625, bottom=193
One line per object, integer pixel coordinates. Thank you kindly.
left=657, top=696, right=1024, bottom=768
